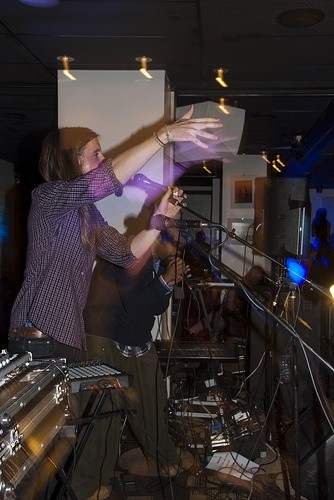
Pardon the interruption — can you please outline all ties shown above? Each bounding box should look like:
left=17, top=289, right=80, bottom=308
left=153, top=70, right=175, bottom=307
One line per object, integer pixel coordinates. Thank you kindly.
left=152, top=255, right=160, bottom=279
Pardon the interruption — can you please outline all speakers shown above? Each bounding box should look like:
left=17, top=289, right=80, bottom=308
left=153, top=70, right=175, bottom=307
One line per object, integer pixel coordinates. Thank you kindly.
left=248, top=287, right=319, bottom=411
left=298, top=435, right=334, bottom=500
left=279, top=404, right=334, bottom=461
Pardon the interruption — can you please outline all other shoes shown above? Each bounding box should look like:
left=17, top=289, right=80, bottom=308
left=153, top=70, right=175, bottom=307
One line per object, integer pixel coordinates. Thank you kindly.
left=162, top=465, right=178, bottom=477
left=184, top=326, right=197, bottom=340
left=86, top=484, right=112, bottom=500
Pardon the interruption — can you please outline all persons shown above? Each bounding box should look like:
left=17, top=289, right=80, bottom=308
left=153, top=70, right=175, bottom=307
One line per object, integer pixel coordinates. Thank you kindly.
left=7, top=103, right=224, bottom=360
left=59, top=226, right=195, bottom=500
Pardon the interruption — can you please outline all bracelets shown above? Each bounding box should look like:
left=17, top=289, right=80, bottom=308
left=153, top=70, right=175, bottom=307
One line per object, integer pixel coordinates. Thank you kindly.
left=164, top=124, right=171, bottom=143
left=153, top=130, right=166, bottom=146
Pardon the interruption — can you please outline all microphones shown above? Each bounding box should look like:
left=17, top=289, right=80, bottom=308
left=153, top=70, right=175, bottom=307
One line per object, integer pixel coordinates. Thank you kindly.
left=134, top=174, right=179, bottom=198
left=151, top=215, right=220, bottom=228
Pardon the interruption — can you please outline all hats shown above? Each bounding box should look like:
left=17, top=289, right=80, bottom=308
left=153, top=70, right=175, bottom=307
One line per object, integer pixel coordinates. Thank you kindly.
left=161, top=228, right=186, bottom=243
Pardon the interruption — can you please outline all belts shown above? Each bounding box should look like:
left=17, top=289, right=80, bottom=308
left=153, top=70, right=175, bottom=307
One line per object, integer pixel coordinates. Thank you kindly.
left=9, top=328, right=50, bottom=339
